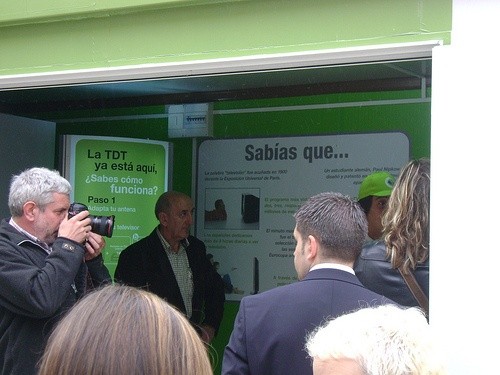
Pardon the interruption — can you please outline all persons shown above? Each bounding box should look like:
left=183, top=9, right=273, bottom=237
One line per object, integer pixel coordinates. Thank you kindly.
left=352, top=158, right=430, bottom=323
left=219, top=192, right=410, bottom=375
left=35, top=283, right=214, bottom=375
left=355, top=172, right=399, bottom=249
left=114, top=191, right=226, bottom=349
left=0, top=167, right=113, bottom=375
left=303, top=304, right=428, bottom=375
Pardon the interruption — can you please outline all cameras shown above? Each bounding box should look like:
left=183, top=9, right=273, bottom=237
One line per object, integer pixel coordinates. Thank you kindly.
left=68, top=203, right=115, bottom=238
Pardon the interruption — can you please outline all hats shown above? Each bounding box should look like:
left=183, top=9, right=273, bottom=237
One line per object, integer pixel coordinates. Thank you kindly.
left=357, top=172, right=398, bottom=201
